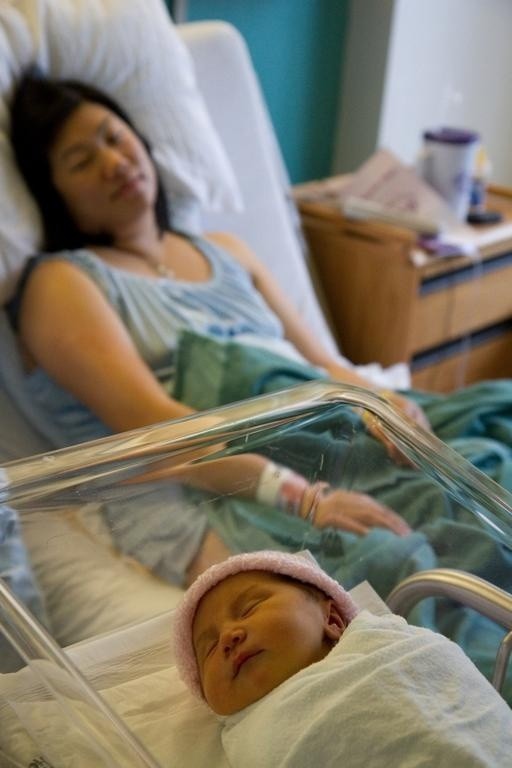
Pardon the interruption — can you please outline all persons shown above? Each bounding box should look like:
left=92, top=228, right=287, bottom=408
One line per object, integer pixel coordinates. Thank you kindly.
left=7, top=59, right=432, bottom=591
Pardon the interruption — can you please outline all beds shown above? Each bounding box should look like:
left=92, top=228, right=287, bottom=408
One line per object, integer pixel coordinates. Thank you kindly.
left=0, top=20, right=511, bottom=709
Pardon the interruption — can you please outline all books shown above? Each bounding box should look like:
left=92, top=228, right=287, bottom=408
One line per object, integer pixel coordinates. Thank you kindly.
left=334, top=147, right=483, bottom=264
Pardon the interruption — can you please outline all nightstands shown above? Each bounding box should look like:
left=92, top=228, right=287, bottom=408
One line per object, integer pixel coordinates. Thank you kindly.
left=293, top=171, right=512, bottom=395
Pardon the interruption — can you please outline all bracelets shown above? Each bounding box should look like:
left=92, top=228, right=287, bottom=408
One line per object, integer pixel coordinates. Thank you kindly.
left=254, top=457, right=326, bottom=527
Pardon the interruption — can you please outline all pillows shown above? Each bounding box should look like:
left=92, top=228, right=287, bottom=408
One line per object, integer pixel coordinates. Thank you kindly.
left=1, top=0, right=243, bottom=308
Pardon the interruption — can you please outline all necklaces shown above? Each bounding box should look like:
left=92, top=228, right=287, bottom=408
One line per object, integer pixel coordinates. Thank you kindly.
left=115, top=235, right=177, bottom=282
